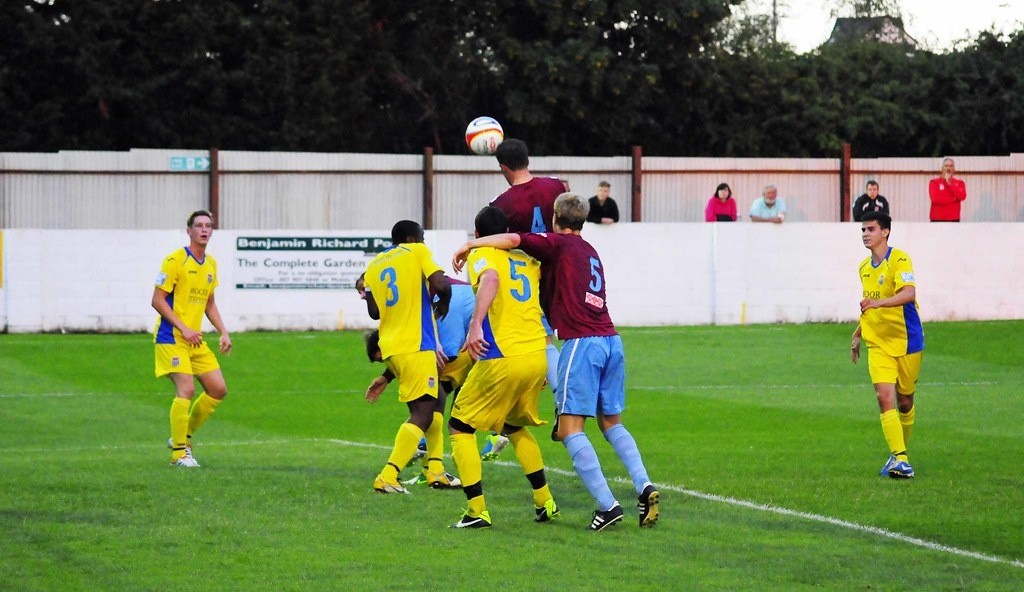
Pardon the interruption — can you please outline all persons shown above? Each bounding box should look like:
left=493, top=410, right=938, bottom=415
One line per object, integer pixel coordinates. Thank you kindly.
left=151, top=209, right=231, bottom=469
left=705, top=183, right=737, bottom=222
left=929, top=157, right=967, bottom=223
left=585, top=180, right=619, bottom=224
left=852, top=181, right=889, bottom=222
left=452, top=189, right=659, bottom=532
left=356, top=140, right=596, bottom=496
left=749, top=185, right=786, bottom=224
left=851, top=212, right=926, bottom=478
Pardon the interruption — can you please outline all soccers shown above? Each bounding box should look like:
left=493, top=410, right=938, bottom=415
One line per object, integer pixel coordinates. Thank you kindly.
left=464, top=115, right=504, bottom=155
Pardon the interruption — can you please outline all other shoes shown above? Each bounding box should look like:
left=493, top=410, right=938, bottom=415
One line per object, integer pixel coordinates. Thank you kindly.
left=550, top=414, right=562, bottom=441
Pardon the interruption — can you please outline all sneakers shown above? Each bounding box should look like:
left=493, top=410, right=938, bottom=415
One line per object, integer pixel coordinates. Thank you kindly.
left=427, top=470, right=462, bottom=488
left=879, top=455, right=897, bottom=476
left=448, top=508, right=491, bottom=530
left=888, top=461, right=915, bottom=478
left=168, top=438, right=192, bottom=456
left=171, top=456, right=199, bottom=468
left=534, top=499, right=561, bottom=523
left=482, top=434, right=509, bottom=463
left=590, top=500, right=624, bottom=531
left=401, top=473, right=427, bottom=485
left=638, top=484, right=660, bottom=528
left=407, top=451, right=424, bottom=468
left=373, top=475, right=410, bottom=493
